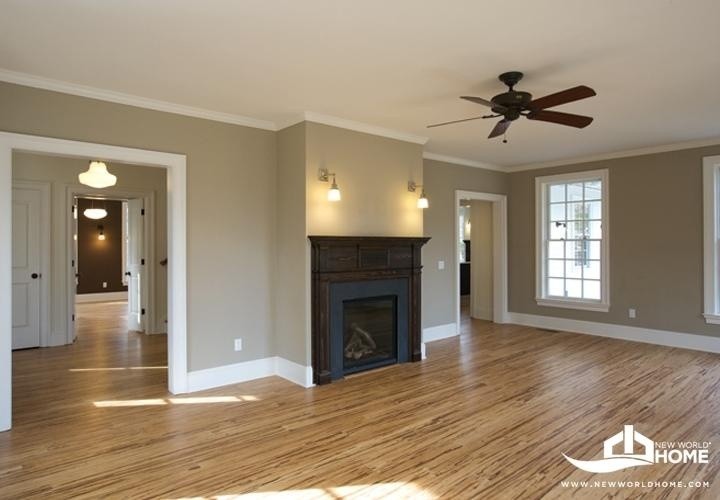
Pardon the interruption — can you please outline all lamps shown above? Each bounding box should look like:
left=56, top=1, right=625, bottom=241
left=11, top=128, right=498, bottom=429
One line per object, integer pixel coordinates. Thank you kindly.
left=97, top=225, right=105, bottom=240
left=319, top=169, right=340, bottom=201
left=408, top=181, right=428, bottom=208
left=79, top=160, right=117, bottom=188
left=83, top=200, right=108, bottom=220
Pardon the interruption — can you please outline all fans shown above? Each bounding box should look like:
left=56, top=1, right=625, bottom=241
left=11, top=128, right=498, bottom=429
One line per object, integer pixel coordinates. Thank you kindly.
left=427, top=72, right=596, bottom=139
